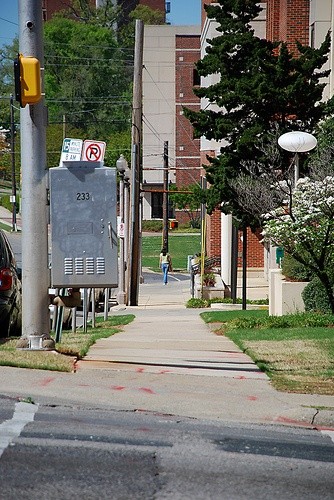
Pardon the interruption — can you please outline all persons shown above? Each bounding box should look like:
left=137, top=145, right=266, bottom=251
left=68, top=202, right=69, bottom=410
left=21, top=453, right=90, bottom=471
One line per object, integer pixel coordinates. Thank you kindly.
left=159, top=248, right=171, bottom=284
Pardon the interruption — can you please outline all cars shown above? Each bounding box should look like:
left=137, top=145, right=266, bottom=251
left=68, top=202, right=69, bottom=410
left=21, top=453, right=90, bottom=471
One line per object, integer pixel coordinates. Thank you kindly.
left=1, top=231, right=21, bottom=342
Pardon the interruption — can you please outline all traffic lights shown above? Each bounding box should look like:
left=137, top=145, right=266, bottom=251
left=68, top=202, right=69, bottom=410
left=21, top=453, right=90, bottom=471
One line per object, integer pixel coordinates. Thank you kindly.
left=167, top=219, right=179, bottom=230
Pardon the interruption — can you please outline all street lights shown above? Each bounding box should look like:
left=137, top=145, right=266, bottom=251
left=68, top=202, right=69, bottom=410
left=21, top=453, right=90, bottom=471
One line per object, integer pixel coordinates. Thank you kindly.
left=278, top=131, right=319, bottom=278
left=116, top=152, right=132, bottom=304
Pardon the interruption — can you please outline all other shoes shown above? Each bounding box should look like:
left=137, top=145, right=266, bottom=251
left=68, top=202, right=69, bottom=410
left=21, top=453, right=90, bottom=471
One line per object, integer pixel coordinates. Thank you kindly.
left=163, top=282, right=168, bottom=285
left=168, top=265, right=172, bottom=272
